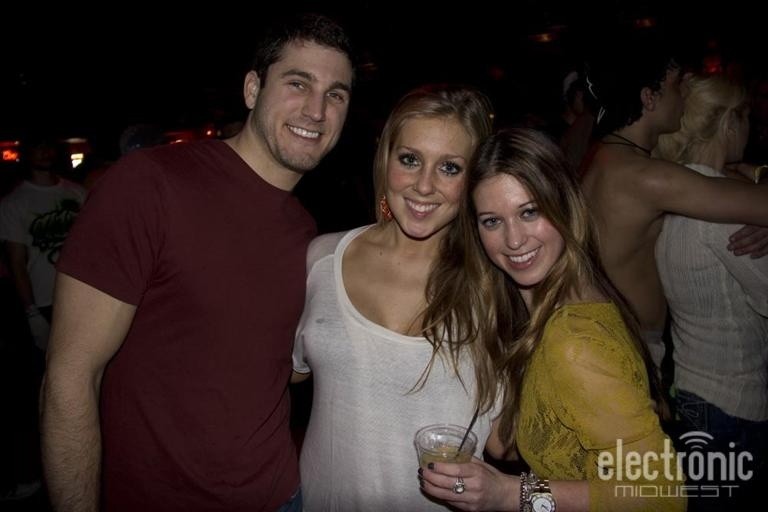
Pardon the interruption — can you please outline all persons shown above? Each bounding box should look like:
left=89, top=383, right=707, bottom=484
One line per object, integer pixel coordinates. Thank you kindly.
left=291, top=77, right=530, bottom=511
left=650, top=68, right=768, bottom=512
left=1, top=125, right=86, bottom=353
left=417, top=124, right=693, bottom=511
left=37, top=17, right=366, bottom=511
left=577, top=14, right=767, bottom=374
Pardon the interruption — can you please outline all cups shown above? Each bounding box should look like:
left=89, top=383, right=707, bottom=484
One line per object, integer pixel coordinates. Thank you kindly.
left=414, top=423, right=478, bottom=467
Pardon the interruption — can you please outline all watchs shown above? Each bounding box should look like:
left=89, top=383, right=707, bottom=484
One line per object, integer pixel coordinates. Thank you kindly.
left=519, top=471, right=557, bottom=512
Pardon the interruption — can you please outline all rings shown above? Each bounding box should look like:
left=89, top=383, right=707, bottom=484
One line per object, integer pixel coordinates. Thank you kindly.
left=453, top=476, right=465, bottom=494
